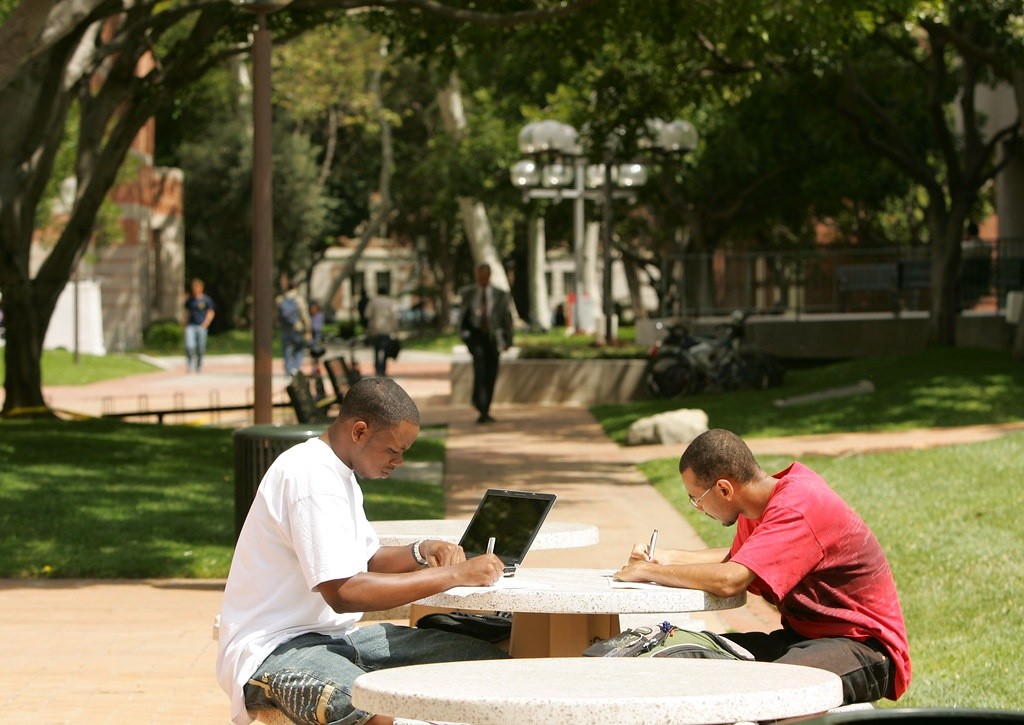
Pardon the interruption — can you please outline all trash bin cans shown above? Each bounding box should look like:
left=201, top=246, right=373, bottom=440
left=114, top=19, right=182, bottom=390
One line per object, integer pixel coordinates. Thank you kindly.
left=234, top=422, right=329, bottom=554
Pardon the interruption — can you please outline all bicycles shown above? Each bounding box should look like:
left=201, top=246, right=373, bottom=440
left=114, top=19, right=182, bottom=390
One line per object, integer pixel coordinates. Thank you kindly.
left=647, top=309, right=753, bottom=397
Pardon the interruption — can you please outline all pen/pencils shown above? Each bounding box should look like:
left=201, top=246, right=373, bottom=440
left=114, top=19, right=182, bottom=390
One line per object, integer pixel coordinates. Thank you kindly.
left=648, top=529, right=658, bottom=560
left=486, top=538, right=496, bottom=554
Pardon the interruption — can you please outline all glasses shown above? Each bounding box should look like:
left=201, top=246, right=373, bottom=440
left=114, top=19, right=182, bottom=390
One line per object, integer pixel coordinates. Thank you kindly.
left=689, top=484, right=716, bottom=507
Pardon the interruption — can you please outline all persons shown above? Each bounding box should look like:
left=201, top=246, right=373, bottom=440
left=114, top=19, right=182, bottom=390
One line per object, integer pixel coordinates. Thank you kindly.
left=457, top=263, right=514, bottom=424
left=364, top=285, right=400, bottom=376
left=358, top=289, right=370, bottom=329
left=277, top=281, right=324, bottom=376
left=181, top=279, right=215, bottom=369
left=216, top=376, right=514, bottom=725
left=614, top=429, right=912, bottom=708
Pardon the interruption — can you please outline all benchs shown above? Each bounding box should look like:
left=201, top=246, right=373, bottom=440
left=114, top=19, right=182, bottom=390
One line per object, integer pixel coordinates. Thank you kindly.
left=322, top=358, right=353, bottom=401
left=285, top=373, right=339, bottom=425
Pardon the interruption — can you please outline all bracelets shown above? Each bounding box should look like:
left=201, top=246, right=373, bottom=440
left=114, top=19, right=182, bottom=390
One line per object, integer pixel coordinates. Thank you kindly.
left=412, top=539, right=428, bottom=566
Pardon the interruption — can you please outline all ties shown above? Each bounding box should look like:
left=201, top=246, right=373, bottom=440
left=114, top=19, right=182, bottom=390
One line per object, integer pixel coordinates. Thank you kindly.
left=480, top=290, right=487, bottom=335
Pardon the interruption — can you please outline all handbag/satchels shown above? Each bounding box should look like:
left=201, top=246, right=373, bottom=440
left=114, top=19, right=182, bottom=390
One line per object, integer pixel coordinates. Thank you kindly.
left=415, top=612, right=512, bottom=643
left=387, top=336, right=402, bottom=359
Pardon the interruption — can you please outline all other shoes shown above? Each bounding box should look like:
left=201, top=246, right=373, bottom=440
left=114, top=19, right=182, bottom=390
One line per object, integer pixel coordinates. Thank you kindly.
left=477, top=411, right=494, bottom=423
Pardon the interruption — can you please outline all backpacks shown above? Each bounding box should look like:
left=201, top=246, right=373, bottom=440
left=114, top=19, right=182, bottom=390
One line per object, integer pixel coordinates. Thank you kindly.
left=581, top=621, right=756, bottom=661
left=277, top=289, right=301, bottom=327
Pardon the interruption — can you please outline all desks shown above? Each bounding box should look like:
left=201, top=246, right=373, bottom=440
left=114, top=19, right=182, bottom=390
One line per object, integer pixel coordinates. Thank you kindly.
left=408, top=567, right=746, bottom=658
left=352, top=656, right=843, bottom=725
left=369, top=520, right=598, bottom=576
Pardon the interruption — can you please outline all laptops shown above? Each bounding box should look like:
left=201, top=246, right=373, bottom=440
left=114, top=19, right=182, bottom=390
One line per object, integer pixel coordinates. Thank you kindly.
left=458, top=488, right=558, bottom=579
